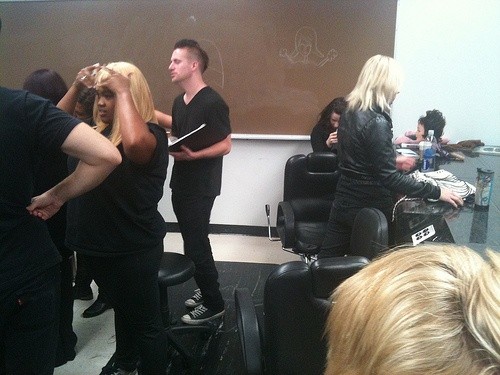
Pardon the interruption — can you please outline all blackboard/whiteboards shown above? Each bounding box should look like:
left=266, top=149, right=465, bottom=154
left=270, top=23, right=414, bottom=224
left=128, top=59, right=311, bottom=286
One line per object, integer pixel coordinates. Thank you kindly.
left=0, top=0, right=398, bottom=141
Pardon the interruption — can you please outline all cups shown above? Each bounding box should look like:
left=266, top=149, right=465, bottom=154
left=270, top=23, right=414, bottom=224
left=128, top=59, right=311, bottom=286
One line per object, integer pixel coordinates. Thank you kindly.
left=419, top=140, right=432, bottom=160
left=474, top=167, right=495, bottom=210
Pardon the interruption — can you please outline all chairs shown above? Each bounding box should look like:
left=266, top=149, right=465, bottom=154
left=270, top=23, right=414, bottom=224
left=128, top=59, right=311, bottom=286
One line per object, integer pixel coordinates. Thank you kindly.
left=235, top=256, right=371, bottom=375
left=265, top=152, right=338, bottom=264
left=351, top=207, right=388, bottom=261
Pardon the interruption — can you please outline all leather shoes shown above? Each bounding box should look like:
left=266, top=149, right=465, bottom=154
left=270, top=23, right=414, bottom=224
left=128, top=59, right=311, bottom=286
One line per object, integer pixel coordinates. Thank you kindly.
left=82, top=295, right=112, bottom=317
left=73, top=286, right=93, bottom=299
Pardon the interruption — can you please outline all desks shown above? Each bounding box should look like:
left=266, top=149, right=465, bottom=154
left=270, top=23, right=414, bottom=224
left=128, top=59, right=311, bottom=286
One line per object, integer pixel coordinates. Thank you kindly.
left=392, top=140, right=500, bottom=251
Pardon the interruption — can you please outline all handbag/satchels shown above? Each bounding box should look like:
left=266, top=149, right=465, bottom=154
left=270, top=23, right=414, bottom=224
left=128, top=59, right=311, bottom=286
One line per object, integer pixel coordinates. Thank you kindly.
left=408, top=169, right=477, bottom=203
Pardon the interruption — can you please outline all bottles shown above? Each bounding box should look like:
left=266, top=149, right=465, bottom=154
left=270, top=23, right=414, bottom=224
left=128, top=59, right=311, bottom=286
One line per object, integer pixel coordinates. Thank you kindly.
left=421, top=130, right=437, bottom=172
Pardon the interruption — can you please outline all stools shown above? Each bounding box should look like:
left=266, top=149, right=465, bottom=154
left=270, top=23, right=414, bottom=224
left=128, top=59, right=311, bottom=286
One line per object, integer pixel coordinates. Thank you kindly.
left=138, top=252, right=211, bottom=371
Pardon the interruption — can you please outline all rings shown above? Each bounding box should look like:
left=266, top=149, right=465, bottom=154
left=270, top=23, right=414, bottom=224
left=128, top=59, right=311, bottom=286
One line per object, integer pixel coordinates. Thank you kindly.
left=80, top=75, right=86, bottom=81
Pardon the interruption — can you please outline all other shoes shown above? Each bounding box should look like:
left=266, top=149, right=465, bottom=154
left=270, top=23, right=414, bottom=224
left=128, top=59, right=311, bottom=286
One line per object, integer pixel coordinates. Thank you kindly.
left=181, top=304, right=225, bottom=324
left=185, top=288, right=204, bottom=307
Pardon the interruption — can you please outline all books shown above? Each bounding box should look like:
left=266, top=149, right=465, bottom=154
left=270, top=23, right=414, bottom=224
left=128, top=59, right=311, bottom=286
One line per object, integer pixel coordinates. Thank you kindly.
left=167, top=124, right=208, bottom=153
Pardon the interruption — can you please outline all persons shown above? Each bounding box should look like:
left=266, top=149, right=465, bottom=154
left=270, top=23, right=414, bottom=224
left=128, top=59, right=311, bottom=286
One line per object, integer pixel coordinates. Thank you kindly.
left=155, top=38, right=234, bottom=324
left=318, top=54, right=464, bottom=259
left=310, top=97, right=347, bottom=153
left=70, top=85, right=114, bottom=318
left=54, top=60, right=170, bottom=374
left=0, top=84, right=122, bottom=375
left=416, top=109, right=445, bottom=142
left=23, top=69, right=68, bottom=106
left=325, top=241, right=499, bottom=375
